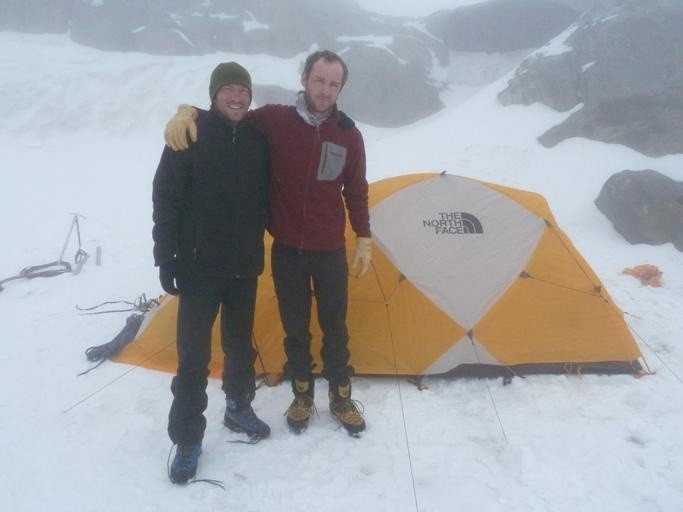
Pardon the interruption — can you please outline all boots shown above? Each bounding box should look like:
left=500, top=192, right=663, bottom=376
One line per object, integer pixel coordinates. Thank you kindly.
left=286, top=376, right=315, bottom=427
left=328, top=379, right=366, bottom=432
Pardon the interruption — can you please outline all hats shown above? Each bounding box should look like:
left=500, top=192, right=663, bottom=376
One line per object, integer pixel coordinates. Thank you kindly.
left=209, top=62, right=253, bottom=106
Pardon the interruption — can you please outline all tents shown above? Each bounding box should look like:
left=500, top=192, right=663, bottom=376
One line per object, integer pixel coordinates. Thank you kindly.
left=107, top=173, right=655, bottom=386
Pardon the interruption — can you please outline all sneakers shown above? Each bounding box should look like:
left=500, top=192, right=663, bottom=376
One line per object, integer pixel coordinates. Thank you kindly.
left=223, top=406, right=270, bottom=439
left=170, top=433, right=207, bottom=482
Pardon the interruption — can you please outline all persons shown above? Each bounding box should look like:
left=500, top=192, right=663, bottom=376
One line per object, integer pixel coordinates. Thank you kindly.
left=161, top=50, right=371, bottom=432
left=151, top=62, right=271, bottom=483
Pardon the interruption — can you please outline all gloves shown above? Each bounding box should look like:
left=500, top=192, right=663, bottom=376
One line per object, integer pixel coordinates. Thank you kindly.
left=159, top=266, right=183, bottom=295
left=350, top=236, right=373, bottom=278
left=163, top=104, right=199, bottom=151
